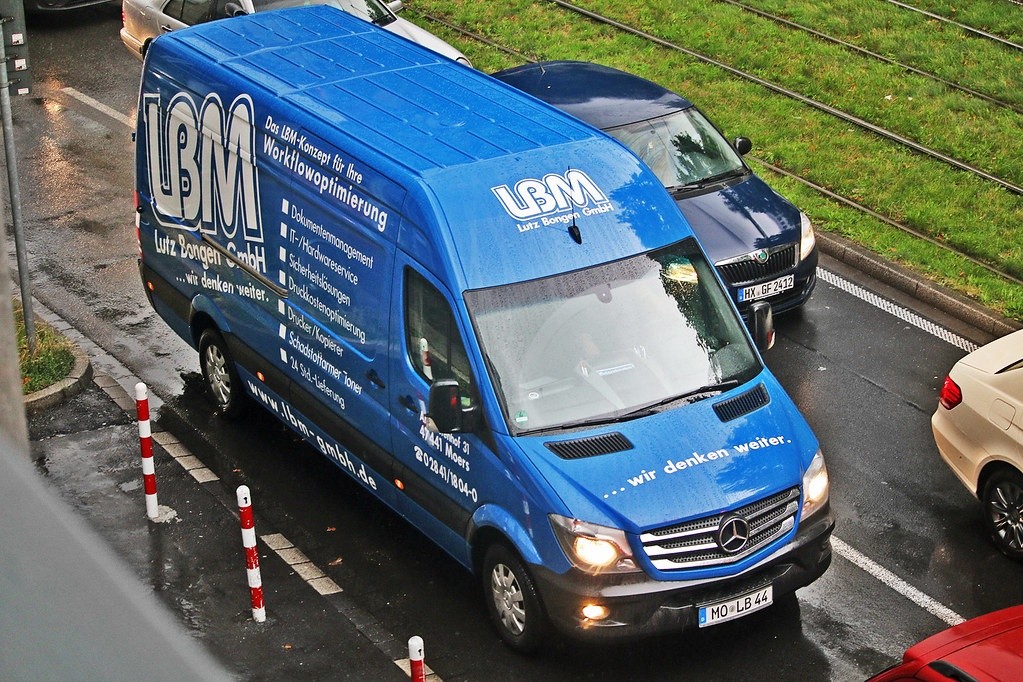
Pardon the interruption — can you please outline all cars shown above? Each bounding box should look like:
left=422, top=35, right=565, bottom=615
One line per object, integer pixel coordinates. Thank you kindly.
left=929, top=326, right=1023, bottom=568
left=118, top=0, right=471, bottom=70
left=870, top=605, right=1022, bottom=682
left=492, top=60, right=822, bottom=324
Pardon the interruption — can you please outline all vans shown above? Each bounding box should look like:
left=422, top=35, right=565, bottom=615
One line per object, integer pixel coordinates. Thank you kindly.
left=138, top=5, right=835, bottom=654
